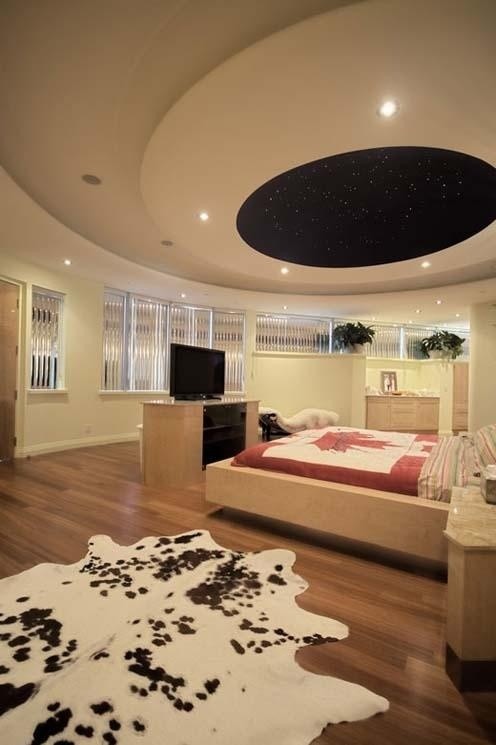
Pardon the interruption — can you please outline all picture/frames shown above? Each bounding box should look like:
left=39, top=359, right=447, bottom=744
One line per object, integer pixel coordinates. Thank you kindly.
left=381, top=371, right=397, bottom=395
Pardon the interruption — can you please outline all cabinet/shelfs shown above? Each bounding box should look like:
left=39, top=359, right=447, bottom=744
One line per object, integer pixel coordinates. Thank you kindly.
left=452, top=362, right=469, bottom=430
left=366, top=395, right=440, bottom=435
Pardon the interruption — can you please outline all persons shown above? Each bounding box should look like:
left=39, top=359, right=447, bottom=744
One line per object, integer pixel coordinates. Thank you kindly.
left=384, top=374, right=395, bottom=392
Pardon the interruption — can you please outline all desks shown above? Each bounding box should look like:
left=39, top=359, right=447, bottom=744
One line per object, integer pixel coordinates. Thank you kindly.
left=139, top=398, right=262, bottom=492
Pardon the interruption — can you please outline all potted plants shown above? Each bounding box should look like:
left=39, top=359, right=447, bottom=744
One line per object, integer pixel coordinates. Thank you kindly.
left=420, top=330, right=465, bottom=359
left=331, top=321, right=376, bottom=354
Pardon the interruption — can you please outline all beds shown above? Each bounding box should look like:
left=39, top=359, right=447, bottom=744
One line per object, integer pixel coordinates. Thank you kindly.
left=205, top=424, right=496, bottom=566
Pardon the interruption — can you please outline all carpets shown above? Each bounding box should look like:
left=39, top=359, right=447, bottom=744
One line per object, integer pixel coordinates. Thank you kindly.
left=1, top=528, right=390, bottom=745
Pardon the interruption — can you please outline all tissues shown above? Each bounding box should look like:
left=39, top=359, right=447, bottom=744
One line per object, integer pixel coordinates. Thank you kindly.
left=479, top=464, right=496, bottom=504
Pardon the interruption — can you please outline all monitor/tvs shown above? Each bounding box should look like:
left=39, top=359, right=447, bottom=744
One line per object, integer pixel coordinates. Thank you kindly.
left=170, top=343, right=225, bottom=401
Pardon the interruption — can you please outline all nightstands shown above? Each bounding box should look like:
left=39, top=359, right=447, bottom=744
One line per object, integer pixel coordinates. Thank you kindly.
left=442, top=487, right=496, bottom=693
